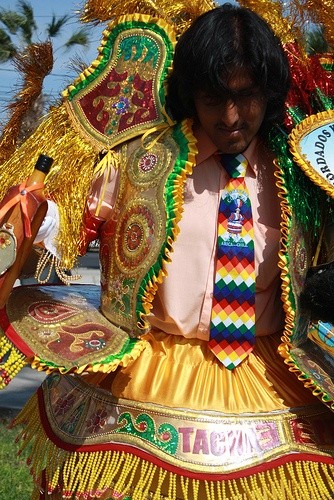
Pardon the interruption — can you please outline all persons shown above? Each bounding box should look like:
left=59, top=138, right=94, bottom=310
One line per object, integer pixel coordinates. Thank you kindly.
left=0, top=5, right=334, bottom=499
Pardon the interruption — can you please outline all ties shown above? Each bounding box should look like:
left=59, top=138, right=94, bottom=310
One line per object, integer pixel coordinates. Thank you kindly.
left=207, top=151, right=256, bottom=373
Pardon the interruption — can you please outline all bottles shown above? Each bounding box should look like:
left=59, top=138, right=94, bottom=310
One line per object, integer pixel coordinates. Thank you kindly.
left=0, top=153, right=54, bottom=310
